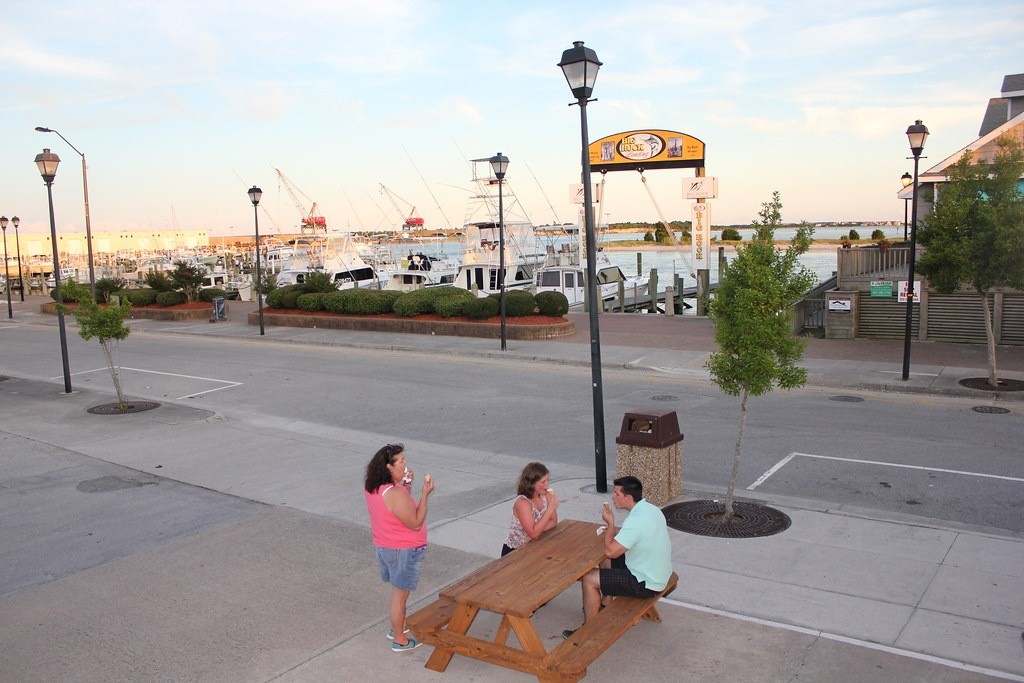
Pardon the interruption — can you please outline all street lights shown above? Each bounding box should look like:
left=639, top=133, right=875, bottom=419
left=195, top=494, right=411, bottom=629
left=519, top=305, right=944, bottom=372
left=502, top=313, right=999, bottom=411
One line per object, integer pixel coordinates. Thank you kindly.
left=33, top=148, right=72, bottom=393
left=0, top=216, right=12, bottom=318
left=557, top=41, right=607, bottom=493
left=902, top=120, right=930, bottom=381
left=12, top=216, right=24, bottom=301
left=246, top=185, right=264, bottom=335
left=489, top=153, right=510, bottom=351
left=900, top=172, right=913, bottom=241
left=35, top=127, right=95, bottom=301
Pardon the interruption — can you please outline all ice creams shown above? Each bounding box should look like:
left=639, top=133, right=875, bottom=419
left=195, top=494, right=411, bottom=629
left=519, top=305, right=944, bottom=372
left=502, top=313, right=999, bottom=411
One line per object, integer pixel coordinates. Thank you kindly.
left=546, top=487, right=554, bottom=498
left=425, top=472, right=431, bottom=482
left=602, top=501, right=609, bottom=511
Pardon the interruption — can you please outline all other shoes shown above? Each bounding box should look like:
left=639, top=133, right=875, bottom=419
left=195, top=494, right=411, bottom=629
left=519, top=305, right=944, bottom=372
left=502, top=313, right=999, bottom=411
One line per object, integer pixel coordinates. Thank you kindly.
left=562, top=630, right=575, bottom=639
left=582, top=603, right=606, bottom=613
left=392, top=638, right=422, bottom=651
left=387, top=625, right=410, bottom=639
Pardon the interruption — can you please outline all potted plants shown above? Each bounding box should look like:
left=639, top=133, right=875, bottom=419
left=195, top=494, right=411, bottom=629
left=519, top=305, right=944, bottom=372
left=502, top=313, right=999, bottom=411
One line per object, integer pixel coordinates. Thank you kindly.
left=841, top=235, right=852, bottom=248
left=877, top=239, right=892, bottom=253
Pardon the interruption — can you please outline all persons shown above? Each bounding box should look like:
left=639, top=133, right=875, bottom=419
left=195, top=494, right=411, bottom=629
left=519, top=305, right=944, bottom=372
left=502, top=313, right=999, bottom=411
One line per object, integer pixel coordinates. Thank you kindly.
left=501, top=462, right=558, bottom=557
left=364, top=445, right=435, bottom=652
left=562, top=475, right=672, bottom=639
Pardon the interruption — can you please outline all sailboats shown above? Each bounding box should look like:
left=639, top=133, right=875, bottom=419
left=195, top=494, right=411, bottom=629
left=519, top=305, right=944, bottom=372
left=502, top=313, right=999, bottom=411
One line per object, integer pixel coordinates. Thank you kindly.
left=0, top=158, right=650, bottom=304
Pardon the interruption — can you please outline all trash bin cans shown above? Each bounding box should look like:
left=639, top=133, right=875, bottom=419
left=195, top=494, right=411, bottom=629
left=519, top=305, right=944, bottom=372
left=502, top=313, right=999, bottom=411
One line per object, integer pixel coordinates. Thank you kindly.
left=615, top=408, right=684, bottom=506
left=824, top=286, right=860, bottom=339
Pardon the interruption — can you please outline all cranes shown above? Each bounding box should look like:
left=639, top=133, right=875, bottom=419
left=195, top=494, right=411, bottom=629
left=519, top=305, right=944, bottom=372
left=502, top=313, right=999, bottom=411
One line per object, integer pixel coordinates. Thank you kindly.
left=275, top=168, right=328, bottom=235
left=378, top=183, right=424, bottom=231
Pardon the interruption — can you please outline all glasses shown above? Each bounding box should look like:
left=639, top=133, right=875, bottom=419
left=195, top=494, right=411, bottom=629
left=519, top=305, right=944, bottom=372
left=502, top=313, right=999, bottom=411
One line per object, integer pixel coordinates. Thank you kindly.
left=387, top=443, right=394, bottom=463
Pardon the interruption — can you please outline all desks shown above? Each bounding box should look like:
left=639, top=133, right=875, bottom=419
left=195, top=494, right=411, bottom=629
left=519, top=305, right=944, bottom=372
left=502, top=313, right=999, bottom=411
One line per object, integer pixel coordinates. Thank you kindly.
left=424, top=519, right=622, bottom=683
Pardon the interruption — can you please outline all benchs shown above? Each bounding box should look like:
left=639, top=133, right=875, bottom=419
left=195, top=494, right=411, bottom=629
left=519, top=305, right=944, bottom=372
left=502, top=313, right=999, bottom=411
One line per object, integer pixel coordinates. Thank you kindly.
left=541, top=573, right=678, bottom=674
left=403, top=601, right=459, bottom=633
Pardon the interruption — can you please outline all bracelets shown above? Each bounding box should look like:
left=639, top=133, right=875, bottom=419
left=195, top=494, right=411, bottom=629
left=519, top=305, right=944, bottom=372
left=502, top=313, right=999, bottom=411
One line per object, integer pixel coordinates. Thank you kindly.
left=404, top=483, right=412, bottom=488
